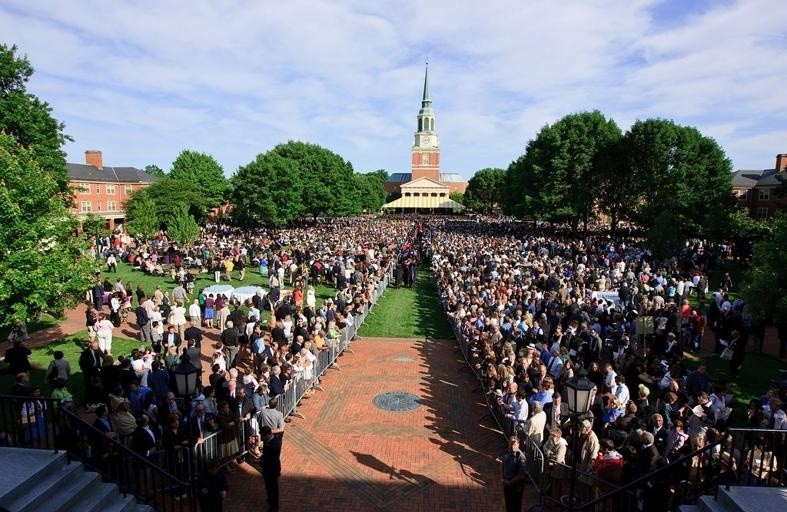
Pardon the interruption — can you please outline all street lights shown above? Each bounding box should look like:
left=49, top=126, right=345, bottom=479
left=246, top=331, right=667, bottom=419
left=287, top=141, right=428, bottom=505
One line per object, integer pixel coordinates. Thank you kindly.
left=562, top=361, right=597, bottom=511
left=92, top=213, right=100, bottom=262
left=169, top=346, right=201, bottom=511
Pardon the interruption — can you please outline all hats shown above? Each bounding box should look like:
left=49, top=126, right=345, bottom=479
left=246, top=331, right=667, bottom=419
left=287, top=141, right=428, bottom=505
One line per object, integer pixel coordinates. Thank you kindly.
left=638, top=384, right=651, bottom=396
left=526, top=343, right=536, bottom=351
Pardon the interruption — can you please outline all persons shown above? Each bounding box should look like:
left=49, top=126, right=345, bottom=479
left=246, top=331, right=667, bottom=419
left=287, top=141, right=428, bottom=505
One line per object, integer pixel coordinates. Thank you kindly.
left=21, top=384, right=47, bottom=449
left=11, top=372, right=33, bottom=444
left=4, top=338, right=32, bottom=375
left=44, top=351, right=71, bottom=392
left=51, top=378, right=76, bottom=429
